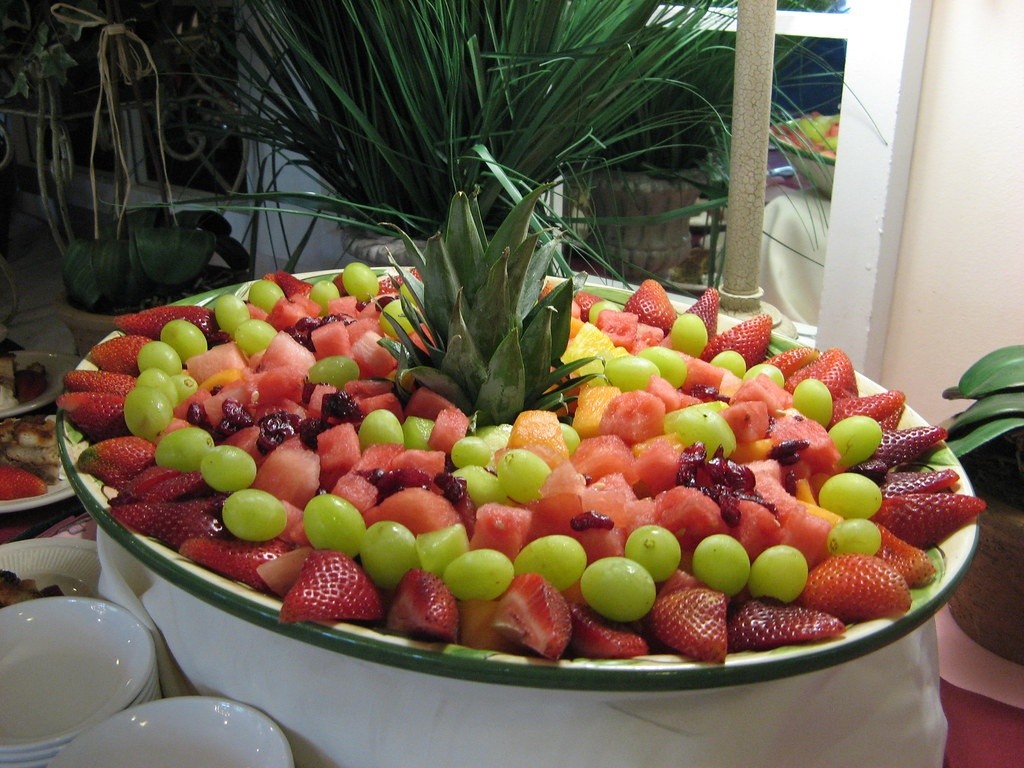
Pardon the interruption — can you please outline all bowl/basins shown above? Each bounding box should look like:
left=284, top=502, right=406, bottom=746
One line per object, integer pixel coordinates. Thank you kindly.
left=768, top=114, right=839, bottom=201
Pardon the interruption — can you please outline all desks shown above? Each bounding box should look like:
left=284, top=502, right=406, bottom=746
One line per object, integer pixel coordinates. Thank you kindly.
left=705, top=148, right=855, bottom=334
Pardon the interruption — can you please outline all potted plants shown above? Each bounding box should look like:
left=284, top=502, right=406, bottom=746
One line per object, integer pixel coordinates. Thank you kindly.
left=945, top=347, right=1024, bottom=666
left=0, top=0, right=837, bottom=350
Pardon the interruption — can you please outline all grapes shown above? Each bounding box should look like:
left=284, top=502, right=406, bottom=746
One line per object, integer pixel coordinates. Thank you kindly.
left=122, top=265, right=886, bottom=622
left=797, top=114, right=838, bottom=151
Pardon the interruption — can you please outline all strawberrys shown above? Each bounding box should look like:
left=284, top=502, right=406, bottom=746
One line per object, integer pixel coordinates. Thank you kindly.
left=776, top=123, right=835, bottom=159
left=0, top=464, right=49, bottom=502
left=57, top=264, right=985, bottom=668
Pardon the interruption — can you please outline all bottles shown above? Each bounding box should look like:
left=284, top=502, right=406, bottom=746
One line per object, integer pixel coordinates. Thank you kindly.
left=0, top=416, right=76, bottom=515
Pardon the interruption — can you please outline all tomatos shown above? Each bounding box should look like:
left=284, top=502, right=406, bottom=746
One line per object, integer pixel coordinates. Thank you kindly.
left=17, top=369, right=48, bottom=402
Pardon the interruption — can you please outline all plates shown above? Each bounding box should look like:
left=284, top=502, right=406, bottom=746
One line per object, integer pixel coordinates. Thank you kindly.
left=0, top=351, right=74, bottom=417
left=0, top=537, right=102, bottom=608
left=43, top=695, right=298, bottom=768
left=0, top=595, right=164, bottom=768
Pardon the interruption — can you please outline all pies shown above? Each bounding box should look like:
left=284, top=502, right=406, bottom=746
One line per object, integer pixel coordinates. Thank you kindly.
left=0, top=417, right=60, bottom=486
left=0, top=352, right=19, bottom=411
